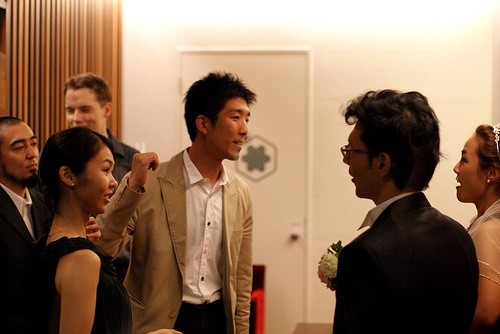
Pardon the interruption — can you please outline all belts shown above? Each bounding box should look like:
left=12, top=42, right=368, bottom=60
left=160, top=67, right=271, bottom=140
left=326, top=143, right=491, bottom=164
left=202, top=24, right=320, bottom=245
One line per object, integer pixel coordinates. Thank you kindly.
left=183, top=300, right=223, bottom=310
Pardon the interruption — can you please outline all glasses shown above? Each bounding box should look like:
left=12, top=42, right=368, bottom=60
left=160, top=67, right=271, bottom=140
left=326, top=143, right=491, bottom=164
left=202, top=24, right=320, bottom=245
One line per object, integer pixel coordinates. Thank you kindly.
left=341, top=145, right=368, bottom=157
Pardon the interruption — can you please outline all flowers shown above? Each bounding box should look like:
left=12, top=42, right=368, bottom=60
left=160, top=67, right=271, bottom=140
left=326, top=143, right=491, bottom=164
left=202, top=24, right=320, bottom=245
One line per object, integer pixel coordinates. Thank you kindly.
left=317, top=240, right=344, bottom=291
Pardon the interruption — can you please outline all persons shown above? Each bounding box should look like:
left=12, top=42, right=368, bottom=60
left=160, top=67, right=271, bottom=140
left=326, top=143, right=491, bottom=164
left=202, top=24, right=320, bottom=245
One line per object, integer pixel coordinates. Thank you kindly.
left=318, top=89, right=479, bottom=334
left=0, top=71, right=259, bottom=334
left=453, top=124, right=500, bottom=334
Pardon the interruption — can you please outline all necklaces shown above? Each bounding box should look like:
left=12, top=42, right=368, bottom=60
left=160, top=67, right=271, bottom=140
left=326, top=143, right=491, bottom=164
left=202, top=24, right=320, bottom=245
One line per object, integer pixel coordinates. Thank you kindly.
left=47, top=230, right=87, bottom=239
